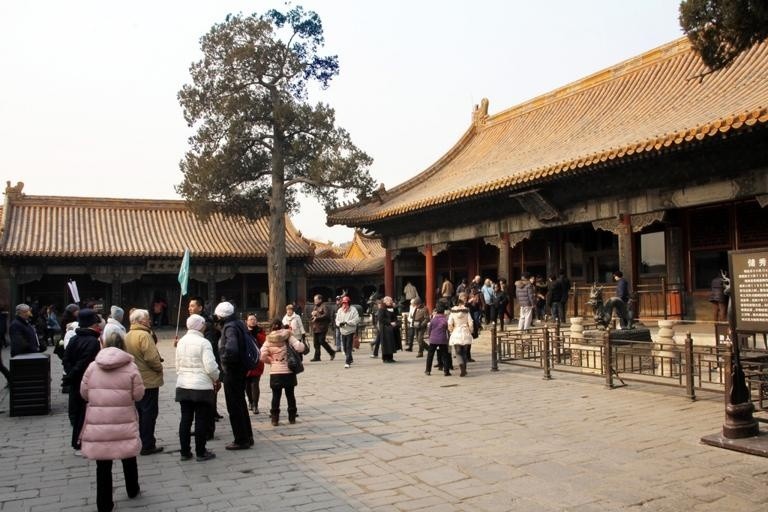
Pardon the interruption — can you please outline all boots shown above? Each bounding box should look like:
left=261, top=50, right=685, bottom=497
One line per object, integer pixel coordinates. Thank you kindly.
left=248, top=399, right=258, bottom=414
left=269, top=407, right=297, bottom=425
left=405, top=344, right=414, bottom=351
left=416, top=351, right=423, bottom=357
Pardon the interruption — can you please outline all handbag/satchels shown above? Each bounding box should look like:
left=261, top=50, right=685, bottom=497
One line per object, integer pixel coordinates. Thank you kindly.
left=412, top=320, right=421, bottom=329
left=301, top=337, right=309, bottom=355
left=286, top=344, right=304, bottom=374
left=490, top=294, right=494, bottom=303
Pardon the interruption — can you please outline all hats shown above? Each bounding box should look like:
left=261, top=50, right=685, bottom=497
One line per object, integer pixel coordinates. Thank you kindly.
left=77, top=309, right=101, bottom=327
left=111, top=305, right=124, bottom=318
left=410, top=296, right=422, bottom=304
left=342, top=296, right=350, bottom=303
left=212, top=302, right=235, bottom=318
left=186, top=314, right=206, bottom=330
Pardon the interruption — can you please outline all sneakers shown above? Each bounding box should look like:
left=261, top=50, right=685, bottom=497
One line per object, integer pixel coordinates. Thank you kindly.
left=225, top=438, right=254, bottom=449
left=345, top=363, right=350, bottom=368
left=369, top=355, right=397, bottom=363
left=180, top=452, right=192, bottom=460
left=197, top=448, right=216, bottom=461
left=310, top=358, right=321, bottom=361
left=330, top=351, right=335, bottom=360
left=425, top=359, right=477, bottom=376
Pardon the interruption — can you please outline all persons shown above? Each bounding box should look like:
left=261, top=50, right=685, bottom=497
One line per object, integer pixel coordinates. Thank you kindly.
left=262, top=319, right=307, bottom=425
left=10, top=304, right=45, bottom=356
left=436, top=275, right=512, bottom=338
left=175, top=294, right=266, bottom=460
left=310, top=295, right=359, bottom=368
left=370, top=296, right=430, bottom=362
left=80, top=333, right=146, bottom=511
left=54, top=296, right=164, bottom=455
left=612, top=270, right=629, bottom=317
left=424, top=300, right=474, bottom=377
left=403, top=282, right=418, bottom=312
left=2, top=305, right=9, bottom=388
left=515, top=269, right=570, bottom=329
left=29, top=301, right=60, bottom=346
left=709, top=273, right=727, bottom=321
left=282, top=304, right=305, bottom=361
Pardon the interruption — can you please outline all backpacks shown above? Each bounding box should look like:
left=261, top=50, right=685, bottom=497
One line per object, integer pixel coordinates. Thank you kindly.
left=220, top=321, right=259, bottom=370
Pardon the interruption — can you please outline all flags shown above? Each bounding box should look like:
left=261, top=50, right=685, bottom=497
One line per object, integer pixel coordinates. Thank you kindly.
left=177, top=248, right=189, bottom=296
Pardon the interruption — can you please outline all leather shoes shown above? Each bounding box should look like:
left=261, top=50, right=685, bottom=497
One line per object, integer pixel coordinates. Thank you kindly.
left=140, top=446, right=164, bottom=455
